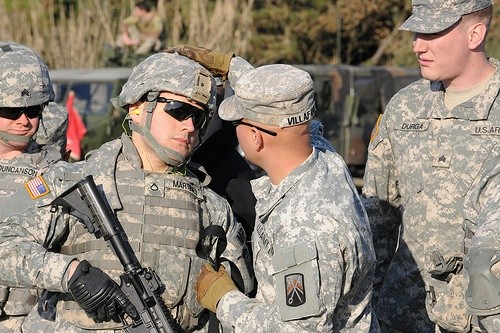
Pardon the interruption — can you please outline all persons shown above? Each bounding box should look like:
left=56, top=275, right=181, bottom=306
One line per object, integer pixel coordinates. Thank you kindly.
left=0, top=41, right=71, bottom=196
left=360, top=0, right=500, bottom=333
left=0, top=52, right=254, bottom=333
left=165, top=44, right=380, bottom=333
left=104, top=2, right=163, bottom=67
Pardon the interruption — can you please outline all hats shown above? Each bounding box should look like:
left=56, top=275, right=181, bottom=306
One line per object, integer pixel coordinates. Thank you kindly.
left=218, top=64, right=317, bottom=129
left=398, top=0, right=494, bottom=33
left=232, top=120, right=277, bottom=136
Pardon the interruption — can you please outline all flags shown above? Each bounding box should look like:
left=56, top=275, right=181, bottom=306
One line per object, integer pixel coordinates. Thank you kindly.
left=65, top=90, right=87, bottom=161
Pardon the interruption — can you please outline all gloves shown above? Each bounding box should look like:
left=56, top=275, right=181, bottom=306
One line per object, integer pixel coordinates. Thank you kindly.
left=195, top=262, right=238, bottom=313
left=67, top=260, right=140, bottom=323
left=166, top=45, right=235, bottom=81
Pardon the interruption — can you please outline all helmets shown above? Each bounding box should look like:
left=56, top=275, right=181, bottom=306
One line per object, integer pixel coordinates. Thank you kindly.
left=110, top=51, right=218, bottom=137
left=0, top=41, right=55, bottom=107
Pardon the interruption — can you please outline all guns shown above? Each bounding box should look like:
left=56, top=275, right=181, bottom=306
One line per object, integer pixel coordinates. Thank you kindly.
left=44, top=174, right=181, bottom=333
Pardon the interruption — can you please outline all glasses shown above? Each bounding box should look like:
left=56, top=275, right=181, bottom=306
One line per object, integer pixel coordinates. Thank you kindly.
left=0, top=105, right=44, bottom=121
left=138, top=93, right=207, bottom=131
